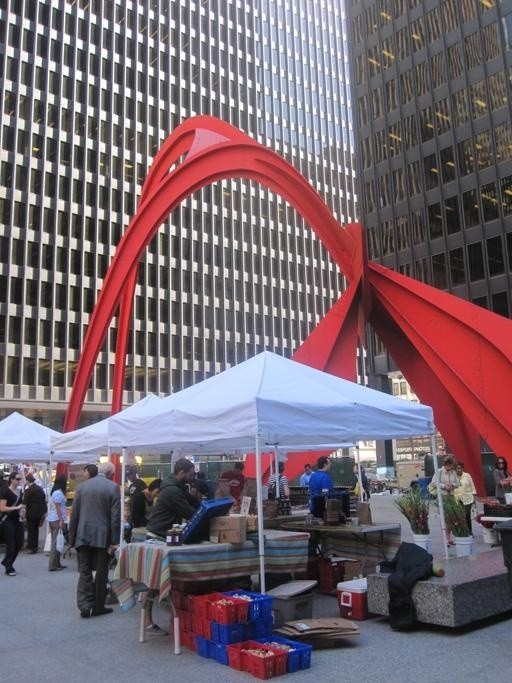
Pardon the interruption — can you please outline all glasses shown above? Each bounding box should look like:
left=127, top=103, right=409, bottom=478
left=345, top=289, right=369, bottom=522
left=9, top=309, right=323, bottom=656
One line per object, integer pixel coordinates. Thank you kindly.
left=12, top=477, right=22, bottom=480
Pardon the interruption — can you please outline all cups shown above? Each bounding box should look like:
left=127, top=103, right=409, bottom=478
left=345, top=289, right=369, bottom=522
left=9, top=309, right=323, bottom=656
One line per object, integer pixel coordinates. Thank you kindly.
left=304, top=514, right=359, bottom=526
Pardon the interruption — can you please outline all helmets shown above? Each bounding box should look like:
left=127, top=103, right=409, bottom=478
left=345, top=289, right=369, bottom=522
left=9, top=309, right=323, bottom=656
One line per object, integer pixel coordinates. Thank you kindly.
left=125, top=468, right=137, bottom=476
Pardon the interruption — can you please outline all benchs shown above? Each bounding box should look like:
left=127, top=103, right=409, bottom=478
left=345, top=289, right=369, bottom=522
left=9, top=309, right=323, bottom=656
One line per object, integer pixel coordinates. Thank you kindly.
left=367, top=550, right=512, bottom=635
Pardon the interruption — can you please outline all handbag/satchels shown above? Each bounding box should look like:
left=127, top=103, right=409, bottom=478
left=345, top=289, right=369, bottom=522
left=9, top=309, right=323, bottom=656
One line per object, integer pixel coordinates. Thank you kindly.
left=426, top=482, right=438, bottom=496
left=267, top=481, right=279, bottom=498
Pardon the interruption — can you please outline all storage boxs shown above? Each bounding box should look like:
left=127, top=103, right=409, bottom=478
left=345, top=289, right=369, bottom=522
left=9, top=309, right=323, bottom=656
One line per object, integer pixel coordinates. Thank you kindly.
left=209, top=515, right=248, bottom=543
left=337, top=578, right=376, bottom=620
left=267, top=592, right=313, bottom=628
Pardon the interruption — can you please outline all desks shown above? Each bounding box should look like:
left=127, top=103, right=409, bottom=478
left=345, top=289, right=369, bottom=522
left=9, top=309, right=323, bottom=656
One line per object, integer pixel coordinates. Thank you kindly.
left=288, top=486, right=353, bottom=505
left=475, top=515, right=512, bottom=527
left=59, top=517, right=76, bottom=560
left=282, top=521, right=402, bottom=577
left=112, top=528, right=312, bottom=654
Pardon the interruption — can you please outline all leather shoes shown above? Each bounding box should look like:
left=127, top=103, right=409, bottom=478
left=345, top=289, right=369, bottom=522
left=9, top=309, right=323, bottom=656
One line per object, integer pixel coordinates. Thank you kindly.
left=49, top=567, right=63, bottom=572
left=57, top=564, right=68, bottom=569
left=91, top=605, right=113, bottom=617
left=79, top=608, right=92, bottom=618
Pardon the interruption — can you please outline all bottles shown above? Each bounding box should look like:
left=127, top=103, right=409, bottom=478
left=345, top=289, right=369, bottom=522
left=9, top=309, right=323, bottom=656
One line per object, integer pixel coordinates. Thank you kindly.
left=19, top=505, right=26, bottom=522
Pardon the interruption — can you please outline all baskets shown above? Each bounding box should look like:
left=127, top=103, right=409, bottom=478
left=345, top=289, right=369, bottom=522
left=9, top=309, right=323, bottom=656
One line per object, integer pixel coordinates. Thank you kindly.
left=195, top=635, right=209, bottom=657
left=249, top=614, right=273, bottom=638
left=220, top=589, right=273, bottom=620
left=210, top=620, right=250, bottom=645
left=253, top=634, right=313, bottom=673
left=225, top=639, right=289, bottom=678
left=169, top=588, right=217, bottom=654
left=209, top=639, right=229, bottom=665
left=209, top=595, right=250, bottom=625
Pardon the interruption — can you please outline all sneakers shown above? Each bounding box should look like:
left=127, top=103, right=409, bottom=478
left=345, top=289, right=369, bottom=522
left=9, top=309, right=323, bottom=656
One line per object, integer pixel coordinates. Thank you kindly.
left=145, top=623, right=169, bottom=637
left=6, top=568, right=17, bottom=577
left=447, top=539, right=456, bottom=548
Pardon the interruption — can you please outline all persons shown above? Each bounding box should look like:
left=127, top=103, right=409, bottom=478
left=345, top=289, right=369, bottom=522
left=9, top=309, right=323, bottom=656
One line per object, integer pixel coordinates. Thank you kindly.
left=452, top=462, right=477, bottom=538
left=494, top=455, right=512, bottom=505
left=307, top=455, right=335, bottom=557
left=139, top=458, right=197, bottom=635
left=221, top=461, right=246, bottom=505
left=268, top=461, right=292, bottom=516
left=298, top=463, right=315, bottom=487
left=355, top=464, right=371, bottom=504
left=0, top=462, right=210, bottom=618
left=430, top=456, right=462, bottom=548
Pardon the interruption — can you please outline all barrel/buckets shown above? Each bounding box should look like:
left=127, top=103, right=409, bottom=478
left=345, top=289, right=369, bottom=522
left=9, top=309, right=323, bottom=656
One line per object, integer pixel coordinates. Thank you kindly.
left=483, top=527, right=499, bottom=543
left=452, top=535, right=474, bottom=558
left=411, top=533, right=431, bottom=551
